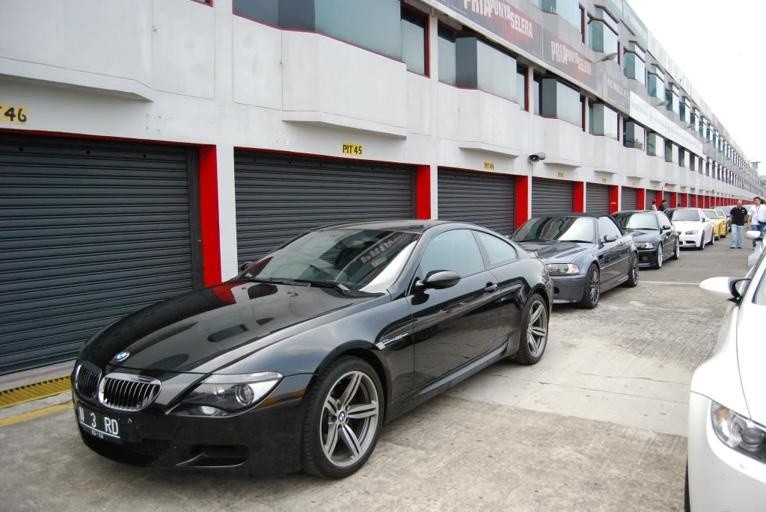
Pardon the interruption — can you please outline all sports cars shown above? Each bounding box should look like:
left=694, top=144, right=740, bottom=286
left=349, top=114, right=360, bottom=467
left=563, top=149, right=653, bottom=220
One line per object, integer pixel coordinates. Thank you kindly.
left=502, top=211, right=639, bottom=309
left=70, top=218, right=555, bottom=480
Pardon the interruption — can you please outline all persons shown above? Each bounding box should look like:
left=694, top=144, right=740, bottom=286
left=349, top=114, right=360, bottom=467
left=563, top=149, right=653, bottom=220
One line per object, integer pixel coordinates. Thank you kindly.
left=651, top=200, right=657, bottom=211
left=730, top=199, right=747, bottom=249
left=747, top=197, right=766, bottom=250
left=659, top=200, right=667, bottom=211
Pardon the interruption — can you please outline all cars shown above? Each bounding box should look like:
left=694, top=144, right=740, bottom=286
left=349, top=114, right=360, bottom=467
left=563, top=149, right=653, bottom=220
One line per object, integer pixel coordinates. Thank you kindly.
left=745, top=225, right=766, bottom=275
left=701, top=204, right=766, bottom=241
left=662, top=208, right=714, bottom=250
left=683, top=244, right=766, bottom=512
left=610, top=210, right=680, bottom=270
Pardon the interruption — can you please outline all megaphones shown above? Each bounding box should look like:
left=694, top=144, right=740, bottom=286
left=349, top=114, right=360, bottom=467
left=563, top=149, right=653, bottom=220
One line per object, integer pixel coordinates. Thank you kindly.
left=528, top=153, right=539, bottom=164
left=537, top=152, right=545, bottom=160
